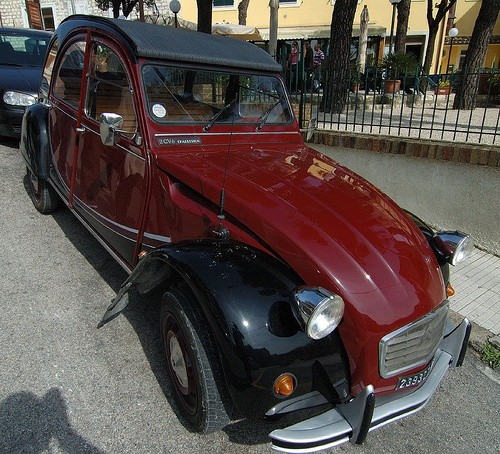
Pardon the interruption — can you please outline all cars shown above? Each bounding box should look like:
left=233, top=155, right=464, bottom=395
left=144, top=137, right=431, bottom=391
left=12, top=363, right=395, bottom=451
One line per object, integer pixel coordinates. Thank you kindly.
left=19, top=12, right=473, bottom=454
left=0, top=26, right=87, bottom=138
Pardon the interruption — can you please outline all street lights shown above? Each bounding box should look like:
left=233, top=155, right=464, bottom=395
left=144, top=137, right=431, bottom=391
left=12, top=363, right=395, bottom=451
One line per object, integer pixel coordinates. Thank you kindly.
left=168, top=0, right=181, bottom=28
left=445, top=28, right=458, bottom=83
left=385, top=0, right=403, bottom=77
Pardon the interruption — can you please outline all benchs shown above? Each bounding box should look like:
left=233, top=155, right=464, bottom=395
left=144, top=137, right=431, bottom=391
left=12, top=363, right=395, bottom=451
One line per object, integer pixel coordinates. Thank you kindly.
left=55, top=77, right=168, bottom=110
left=90, top=97, right=214, bottom=138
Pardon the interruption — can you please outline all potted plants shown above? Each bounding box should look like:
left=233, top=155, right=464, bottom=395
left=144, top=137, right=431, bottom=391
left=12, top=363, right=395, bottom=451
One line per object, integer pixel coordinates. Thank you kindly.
left=290, top=96, right=318, bottom=120
left=434, top=78, right=453, bottom=94
left=379, top=52, right=419, bottom=94
left=352, top=80, right=360, bottom=91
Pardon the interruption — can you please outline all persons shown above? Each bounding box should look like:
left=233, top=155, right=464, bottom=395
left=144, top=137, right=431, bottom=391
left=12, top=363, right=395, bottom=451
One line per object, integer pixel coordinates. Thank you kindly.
left=288, top=40, right=325, bottom=94
